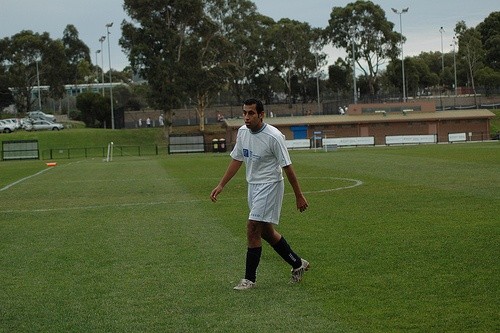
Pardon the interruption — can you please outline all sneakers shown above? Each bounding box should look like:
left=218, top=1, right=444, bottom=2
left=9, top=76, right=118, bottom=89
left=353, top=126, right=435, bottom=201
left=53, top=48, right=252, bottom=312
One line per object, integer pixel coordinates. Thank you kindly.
left=289, top=258, right=310, bottom=285
left=233, top=279, right=256, bottom=290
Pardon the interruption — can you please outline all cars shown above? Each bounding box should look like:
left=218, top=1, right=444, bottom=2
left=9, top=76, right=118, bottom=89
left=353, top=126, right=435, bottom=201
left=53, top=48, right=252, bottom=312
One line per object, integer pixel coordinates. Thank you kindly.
left=0, top=111, right=64, bottom=133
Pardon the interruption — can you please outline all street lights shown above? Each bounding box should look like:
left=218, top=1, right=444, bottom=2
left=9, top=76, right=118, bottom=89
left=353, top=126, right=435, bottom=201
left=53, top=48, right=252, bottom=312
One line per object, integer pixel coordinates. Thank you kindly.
left=35, top=56, right=42, bottom=111
left=348, top=24, right=358, bottom=106
left=439, top=26, right=445, bottom=95
left=391, top=8, right=409, bottom=102
left=99, top=35, right=107, bottom=129
left=96, top=49, right=101, bottom=85
left=106, top=22, right=118, bottom=130
left=309, top=41, right=322, bottom=115
left=450, top=43, right=459, bottom=96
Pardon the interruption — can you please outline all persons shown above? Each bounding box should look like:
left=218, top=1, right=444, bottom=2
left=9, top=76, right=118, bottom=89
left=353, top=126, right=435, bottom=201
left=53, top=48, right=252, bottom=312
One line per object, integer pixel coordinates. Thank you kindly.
left=146, top=117, right=151, bottom=128
left=338, top=105, right=348, bottom=115
left=139, top=118, right=143, bottom=127
left=216, top=111, right=224, bottom=123
left=158, top=114, right=164, bottom=128
left=210, top=98, right=310, bottom=291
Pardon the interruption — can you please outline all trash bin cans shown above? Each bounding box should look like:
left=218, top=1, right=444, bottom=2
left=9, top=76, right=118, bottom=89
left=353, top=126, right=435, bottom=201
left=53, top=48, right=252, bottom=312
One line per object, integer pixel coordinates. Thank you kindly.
left=310, top=131, right=323, bottom=152
left=219, top=138, right=226, bottom=152
left=212, top=139, right=219, bottom=152
